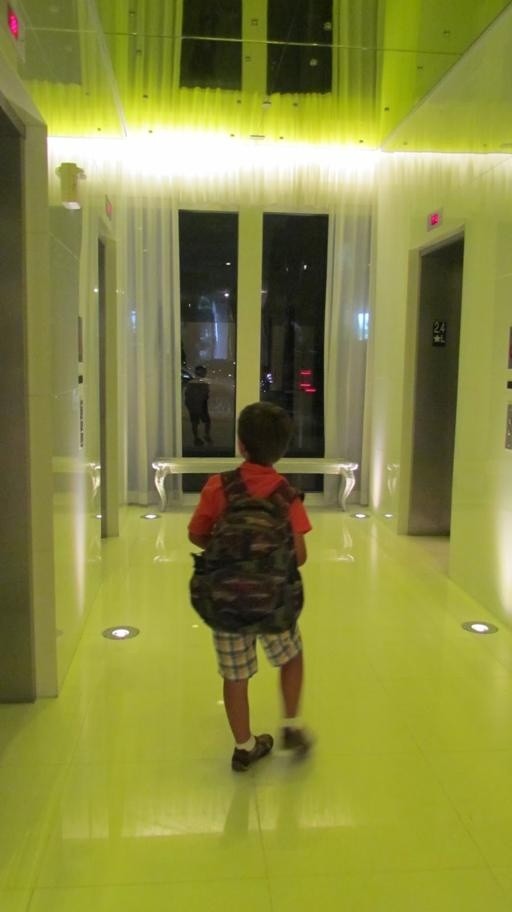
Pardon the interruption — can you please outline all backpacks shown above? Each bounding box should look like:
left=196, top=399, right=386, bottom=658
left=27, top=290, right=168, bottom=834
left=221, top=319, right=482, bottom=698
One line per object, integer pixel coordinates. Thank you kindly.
left=178, top=469, right=308, bottom=636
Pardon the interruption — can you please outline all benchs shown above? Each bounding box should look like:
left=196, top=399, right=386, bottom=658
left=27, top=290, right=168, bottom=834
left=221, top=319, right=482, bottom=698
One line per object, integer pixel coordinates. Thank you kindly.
left=153, top=455, right=361, bottom=513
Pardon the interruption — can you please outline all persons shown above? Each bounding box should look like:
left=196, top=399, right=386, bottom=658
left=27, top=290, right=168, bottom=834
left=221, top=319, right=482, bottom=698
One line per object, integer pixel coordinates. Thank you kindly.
left=183, top=364, right=215, bottom=445
left=186, top=403, right=313, bottom=774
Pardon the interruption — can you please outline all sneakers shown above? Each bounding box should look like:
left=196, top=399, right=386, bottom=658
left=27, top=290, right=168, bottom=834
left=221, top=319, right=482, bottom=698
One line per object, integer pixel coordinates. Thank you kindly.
left=280, top=724, right=318, bottom=752
left=228, top=732, right=275, bottom=772
left=192, top=435, right=212, bottom=445
